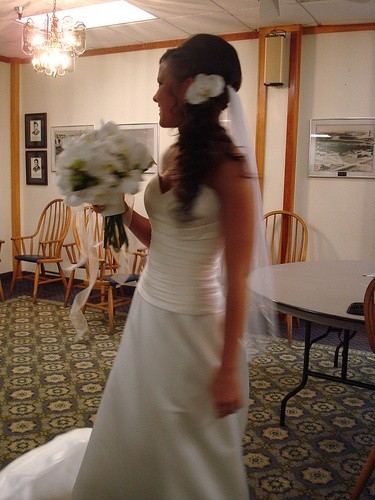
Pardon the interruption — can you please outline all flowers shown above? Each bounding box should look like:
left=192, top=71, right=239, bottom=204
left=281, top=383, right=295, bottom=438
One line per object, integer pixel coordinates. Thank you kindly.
left=184, top=73, right=225, bottom=105
left=53, top=120, right=159, bottom=252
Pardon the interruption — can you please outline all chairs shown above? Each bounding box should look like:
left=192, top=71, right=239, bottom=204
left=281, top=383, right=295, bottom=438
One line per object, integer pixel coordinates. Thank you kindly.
left=351, top=277, right=375, bottom=500
left=9, top=198, right=71, bottom=303
left=263, top=211, right=308, bottom=343
left=62, top=206, right=124, bottom=314
left=78, top=248, right=149, bottom=334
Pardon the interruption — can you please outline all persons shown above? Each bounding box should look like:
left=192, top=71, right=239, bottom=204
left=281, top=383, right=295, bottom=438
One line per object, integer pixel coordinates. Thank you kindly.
left=58, top=33, right=273, bottom=500
left=31, top=122, right=40, bottom=135
left=32, top=159, right=40, bottom=172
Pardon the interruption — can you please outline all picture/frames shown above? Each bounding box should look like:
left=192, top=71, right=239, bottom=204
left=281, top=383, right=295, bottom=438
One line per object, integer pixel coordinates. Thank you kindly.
left=25, top=150, right=48, bottom=185
left=51, top=123, right=94, bottom=172
left=25, top=113, right=47, bottom=149
left=308, top=117, right=375, bottom=179
left=116, top=124, right=158, bottom=175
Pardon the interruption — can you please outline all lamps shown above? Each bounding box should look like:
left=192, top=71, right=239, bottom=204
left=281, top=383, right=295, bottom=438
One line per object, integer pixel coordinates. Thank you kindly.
left=20, top=0, right=86, bottom=77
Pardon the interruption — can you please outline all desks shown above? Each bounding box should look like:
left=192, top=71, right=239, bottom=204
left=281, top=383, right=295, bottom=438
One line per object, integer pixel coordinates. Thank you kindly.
left=246, top=261, right=375, bottom=426
